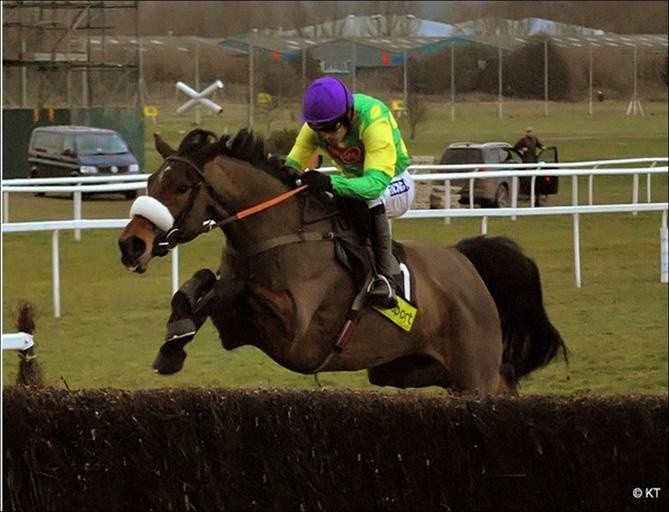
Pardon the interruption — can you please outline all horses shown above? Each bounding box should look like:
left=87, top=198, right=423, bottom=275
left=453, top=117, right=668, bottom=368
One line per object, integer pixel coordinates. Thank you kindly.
left=118, top=127, right=569, bottom=400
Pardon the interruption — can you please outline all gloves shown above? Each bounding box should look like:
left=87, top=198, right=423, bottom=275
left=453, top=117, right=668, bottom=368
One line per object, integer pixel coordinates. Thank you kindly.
left=301, top=170, right=332, bottom=192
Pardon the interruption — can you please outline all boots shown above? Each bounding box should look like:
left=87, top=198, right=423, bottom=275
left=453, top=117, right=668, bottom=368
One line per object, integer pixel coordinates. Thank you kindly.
left=368, top=212, right=398, bottom=308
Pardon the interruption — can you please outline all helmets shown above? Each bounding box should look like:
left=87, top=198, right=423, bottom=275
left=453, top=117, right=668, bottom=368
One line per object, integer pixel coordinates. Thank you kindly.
left=301, top=78, right=355, bottom=122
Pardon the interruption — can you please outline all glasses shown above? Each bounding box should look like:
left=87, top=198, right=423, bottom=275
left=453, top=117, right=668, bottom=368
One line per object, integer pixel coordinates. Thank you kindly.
left=307, top=119, right=346, bottom=134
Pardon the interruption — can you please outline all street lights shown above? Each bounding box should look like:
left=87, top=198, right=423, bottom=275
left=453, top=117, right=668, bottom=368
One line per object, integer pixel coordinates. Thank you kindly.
left=246, top=26, right=258, bottom=128
left=588, top=28, right=604, bottom=115
left=403, top=14, right=417, bottom=108
left=346, top=14, right=356, bottom=94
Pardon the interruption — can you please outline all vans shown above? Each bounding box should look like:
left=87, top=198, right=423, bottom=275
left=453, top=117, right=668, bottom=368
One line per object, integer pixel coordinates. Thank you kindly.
left=27, top=125, right=140, bottom=200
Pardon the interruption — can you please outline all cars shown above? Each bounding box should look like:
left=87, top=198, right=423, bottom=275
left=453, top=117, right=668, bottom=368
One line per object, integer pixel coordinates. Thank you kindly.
left=440, top=141, right=559, bottom=208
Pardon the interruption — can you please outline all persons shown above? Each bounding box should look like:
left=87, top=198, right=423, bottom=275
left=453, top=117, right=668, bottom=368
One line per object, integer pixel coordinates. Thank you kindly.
left=513, top=126, right=547, bottom=205
left=283, top=74, right=417, bottom=297
